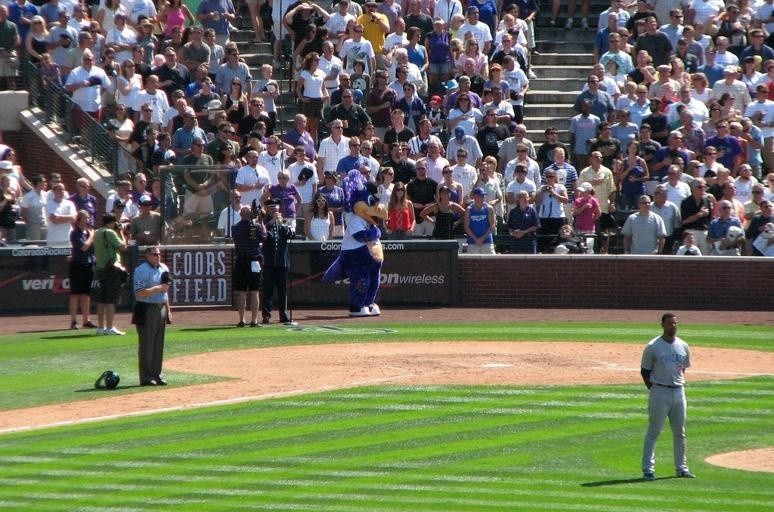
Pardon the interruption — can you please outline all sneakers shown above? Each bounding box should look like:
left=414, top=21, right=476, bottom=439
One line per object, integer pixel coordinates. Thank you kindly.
left=69, top=321, right=126, bottom=337
left=642, top=473, right=655, bottom=482
left=234, top=316, right=298, bottom=328
left=349, top=304, right=382, bottom=318
left=677, top=471, right=696, bottom=478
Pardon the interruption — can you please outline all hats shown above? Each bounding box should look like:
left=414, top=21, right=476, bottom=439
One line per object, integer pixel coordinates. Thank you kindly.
left=722, top=64, right=738, bottom=73
left=433, top=18, right=445, bottom=25
left=576, top=182, right=592, bottom=192
left=361, top=2, right=382, bottom=7
left=490, top=63, right=502, bottom=69
left=263, top=199, right=281, bottom=210
left=298, top=168, right=314, bottom=181
left=472, top=187, right=487, bottom=195
left=454, top=126, right=465, bottom=140
left=138, top=196, right=152, bottom=205
left=324, top=171, right=338, bottom=182
left=136, top=19, right=154, bottom=30
left=508, top=27, right=519, bottom=34
left=113, top=199, right=125, bottom=208
left=0, top=161, right=13, bottom=171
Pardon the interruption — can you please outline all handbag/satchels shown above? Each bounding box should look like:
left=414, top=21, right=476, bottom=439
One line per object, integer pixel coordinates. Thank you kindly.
left=104, top=261, right=128, bottom=285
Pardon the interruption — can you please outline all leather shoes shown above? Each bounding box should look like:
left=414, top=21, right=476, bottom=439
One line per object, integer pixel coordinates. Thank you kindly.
left=136, top=378, right=167, bottom=386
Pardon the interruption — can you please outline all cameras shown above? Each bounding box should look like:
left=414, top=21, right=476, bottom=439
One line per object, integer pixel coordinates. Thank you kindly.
left=544, top=186, right=550, bottom=190
left=114, top=222, right=124, bottom=229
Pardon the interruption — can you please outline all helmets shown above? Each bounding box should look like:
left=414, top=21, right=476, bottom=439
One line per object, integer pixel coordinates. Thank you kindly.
left=96, top=368, right=120, bottom=391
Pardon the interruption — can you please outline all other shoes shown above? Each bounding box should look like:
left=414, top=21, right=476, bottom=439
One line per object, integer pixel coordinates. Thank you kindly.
left=565, top=22, right=572, bottom=29
left=532, top=51, right=539, bottom=55
left=582, top=22, right=588, bottom=29
left=528, top=71, right=536, bottom=78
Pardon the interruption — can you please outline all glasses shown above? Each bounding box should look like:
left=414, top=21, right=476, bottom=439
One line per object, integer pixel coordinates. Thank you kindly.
left=148, top=252, right=161, bottom=256
left=31, top=0, right=773, bottom=212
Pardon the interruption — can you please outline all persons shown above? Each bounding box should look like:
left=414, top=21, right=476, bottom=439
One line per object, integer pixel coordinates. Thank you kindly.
left=620, top=195, right=666, bottom=254
left=130, top=247, right=172, bottom=386
left=260, top=198, right=295, bottom=325
left=3, top=1, right=772, bottom=245
left=93, top=213, right=129, bottom=336
left=68, top=210, right=96, bottom=330
left=68, top=209, right=98, bottom=329
left=676, top=230, right=701, bottom=255
left=709, top=226, right=745, bottom=256
left=640, top=313, right=695, bottom=480
left=507, top=190, right=539, bottom=254
left=463, top=188, right=496, bottom=255
left=572, top=182, right=600, bottom=253
left=753, top=223, right=772, bottom=256
left=553, top=233, right=570, bottom=254
left=230, top=205, right=268, bottom=327
